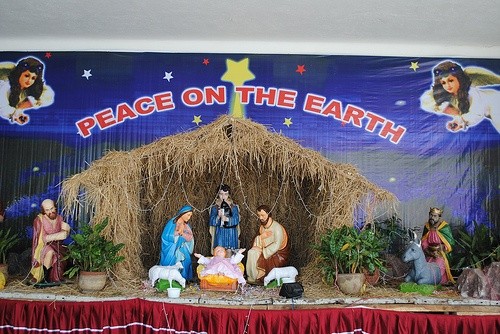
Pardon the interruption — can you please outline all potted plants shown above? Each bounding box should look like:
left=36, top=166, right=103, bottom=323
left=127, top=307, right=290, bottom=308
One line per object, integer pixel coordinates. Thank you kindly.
left=311, top=214, right=416, bottom=296
left=58, top=217, right=126, bottom=293
left=0, top=227, right=20, bottom=279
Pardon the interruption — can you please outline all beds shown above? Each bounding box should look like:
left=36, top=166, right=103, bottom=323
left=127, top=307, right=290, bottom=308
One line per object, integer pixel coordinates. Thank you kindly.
left=197, top=248, right=244, bottom=292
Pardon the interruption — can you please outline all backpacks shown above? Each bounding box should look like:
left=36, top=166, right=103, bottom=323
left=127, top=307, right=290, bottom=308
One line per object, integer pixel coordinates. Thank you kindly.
left=279, top=281, right=305, bottom=301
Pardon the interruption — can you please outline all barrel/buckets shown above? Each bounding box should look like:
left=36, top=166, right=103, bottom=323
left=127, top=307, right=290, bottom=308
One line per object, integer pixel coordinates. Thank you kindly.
left=167, top=288, right=182, bottom=298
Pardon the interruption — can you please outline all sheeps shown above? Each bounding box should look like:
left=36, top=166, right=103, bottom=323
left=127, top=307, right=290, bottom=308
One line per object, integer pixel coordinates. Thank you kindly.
left=149, top=265, right=186, bottom=289
left=263, top=267, right=298, bottom=287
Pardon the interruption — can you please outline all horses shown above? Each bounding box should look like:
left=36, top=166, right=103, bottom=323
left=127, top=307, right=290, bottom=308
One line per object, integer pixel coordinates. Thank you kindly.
left=402, top=229, right=448, bottom=286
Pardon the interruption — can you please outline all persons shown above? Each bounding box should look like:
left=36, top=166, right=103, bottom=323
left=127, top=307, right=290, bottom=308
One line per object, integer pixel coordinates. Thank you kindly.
left=209, top=185, right=240, bottom=255
left=420, top=61, right=500, bottom=135
left=420, top=207, right=455, bottom=286
left=0, top=57, right=45, bottom=126
left=29, top=199, right=71, bottom=282
left=246, top=206, right=289, bottom=281
left=159, top=204, right=195, bottom=282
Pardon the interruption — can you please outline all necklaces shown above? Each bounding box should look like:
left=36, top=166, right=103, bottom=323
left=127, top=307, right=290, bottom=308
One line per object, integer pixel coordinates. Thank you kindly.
left=263, top=219, right=273, bottom=229
